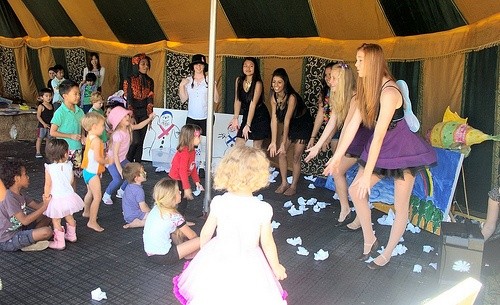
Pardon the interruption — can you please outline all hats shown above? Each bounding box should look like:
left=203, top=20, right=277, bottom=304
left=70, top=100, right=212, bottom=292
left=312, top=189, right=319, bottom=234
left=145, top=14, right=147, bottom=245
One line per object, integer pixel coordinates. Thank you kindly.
left=131, top=53, right=152, bottom=75
left=107, top=106, right=133, bottom=131
left=188, top=54, right=209, bottom=72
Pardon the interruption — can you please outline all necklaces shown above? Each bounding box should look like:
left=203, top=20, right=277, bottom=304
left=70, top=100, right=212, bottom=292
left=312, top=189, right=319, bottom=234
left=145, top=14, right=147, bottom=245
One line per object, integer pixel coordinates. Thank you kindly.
left=276, top=93, right=286, bottom=110
left=245, top=79, right=252, bottom=91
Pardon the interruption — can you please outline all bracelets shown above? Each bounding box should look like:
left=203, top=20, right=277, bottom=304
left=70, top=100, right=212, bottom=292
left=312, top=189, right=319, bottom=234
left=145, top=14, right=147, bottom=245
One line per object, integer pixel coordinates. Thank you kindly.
left=310, top=136, right=315, bottom=138
left=247, top=124, right=250, bottom=128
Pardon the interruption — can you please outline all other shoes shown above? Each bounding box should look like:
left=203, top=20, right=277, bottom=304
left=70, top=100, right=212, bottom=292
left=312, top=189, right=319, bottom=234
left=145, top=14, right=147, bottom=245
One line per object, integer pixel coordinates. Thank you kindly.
left=358, top=239, right=379, bottom=262
left=367, top=259, right=390, bottom=271
left=275, top=182, right=290, bottom=194
left=340, top=224, right=362, bottom=232
left=283, top=187, right=297, bottom=197
left=115, top=189, right=123, bottom=199
left=335, top=211, right=352, bottom=226
left=35, top=153, right=43, bottom=158
left=20, top=239, right=49, bottom=253
left=102, top=192, right=114, bottom=204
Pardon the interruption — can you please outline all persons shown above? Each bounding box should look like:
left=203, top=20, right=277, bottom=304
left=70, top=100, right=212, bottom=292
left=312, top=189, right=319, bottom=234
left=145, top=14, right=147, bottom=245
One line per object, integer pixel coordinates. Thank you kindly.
left=37, top=52, right=156, bottom=204
left=322, top=43, right=438, bottom=270
left=44, top=139, right=86, bottom=250
left=169, top=124, right=204, bottom=226
left=179, top=54, right=219, bottom=136
left=267, top=67, right=314, bottom=196
left=173, top=146, right=288, bottom=305
left=228, top=57, right=271, bottom=146
left=0, top=159, right=53, bottom=251
left=121, top=162, right=151, bottom=228
left=304, top=63, right=369, bottom=232
left=143, top=176, right=201, bottom=265
left=481, top=186, right=500, bottom=275
left=82, top=112, right=109, bottom=231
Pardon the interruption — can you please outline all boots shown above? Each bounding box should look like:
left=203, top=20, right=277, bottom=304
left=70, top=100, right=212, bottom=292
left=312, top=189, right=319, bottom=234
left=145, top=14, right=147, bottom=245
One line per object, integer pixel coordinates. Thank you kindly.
left=64, top=221, right=78, bottom=243
left=46, top=226, right=66, bottom=250
